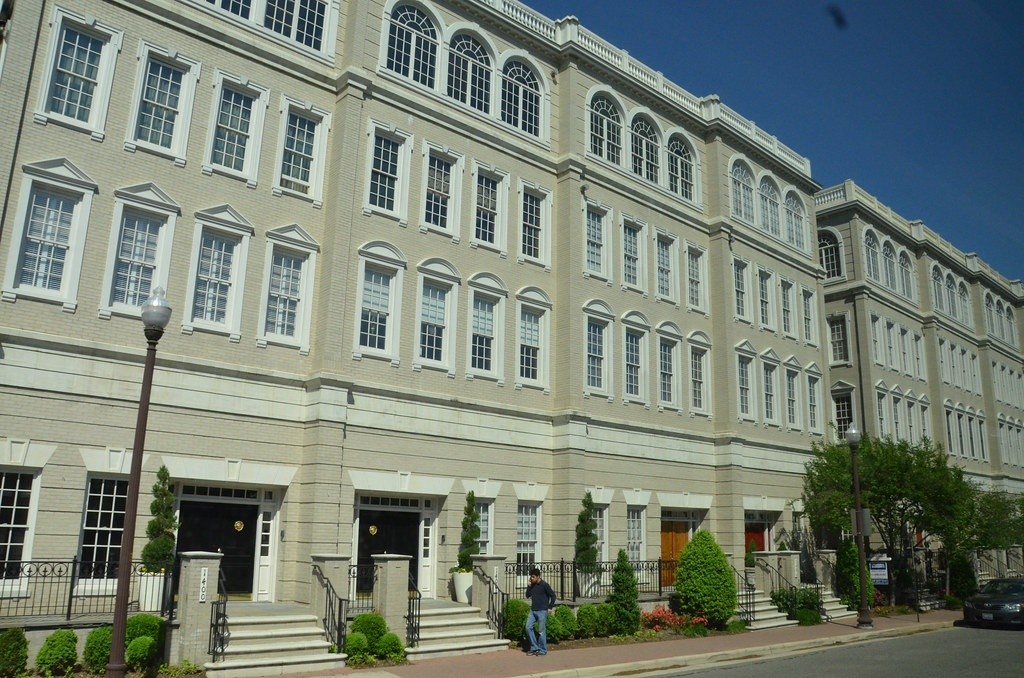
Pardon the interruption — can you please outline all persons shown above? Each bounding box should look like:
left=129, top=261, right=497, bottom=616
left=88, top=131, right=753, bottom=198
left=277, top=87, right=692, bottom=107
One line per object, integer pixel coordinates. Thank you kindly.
left=526, top=569, right=556, bottom=655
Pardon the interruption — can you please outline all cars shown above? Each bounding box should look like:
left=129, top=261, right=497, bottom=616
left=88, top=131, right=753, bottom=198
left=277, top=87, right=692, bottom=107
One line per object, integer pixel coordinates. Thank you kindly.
left=962, top=578, right=1024, bottom=627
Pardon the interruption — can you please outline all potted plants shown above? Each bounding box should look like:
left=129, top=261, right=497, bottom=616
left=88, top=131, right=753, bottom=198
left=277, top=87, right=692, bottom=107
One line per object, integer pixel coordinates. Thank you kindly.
left=448, top=490, right=482, bottom=604
left=571, top=489, right=602, bottom=598
left=136, top=464, right=184, bottom=612
left=744, top=539, right=760, bottom=584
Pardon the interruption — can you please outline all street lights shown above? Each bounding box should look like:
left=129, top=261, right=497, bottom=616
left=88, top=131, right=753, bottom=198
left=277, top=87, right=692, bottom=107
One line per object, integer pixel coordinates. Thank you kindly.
left=845, top=420, right=876, bottom=627
left=102, top=287, right=175, bottom=678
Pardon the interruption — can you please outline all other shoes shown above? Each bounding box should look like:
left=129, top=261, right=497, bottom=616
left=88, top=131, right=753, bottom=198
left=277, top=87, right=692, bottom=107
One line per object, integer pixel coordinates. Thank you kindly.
left=538, top=650, right=546, bottom=655
left=527, top=650, right=538, bottom=655
